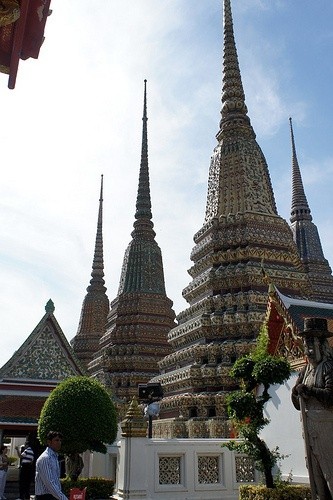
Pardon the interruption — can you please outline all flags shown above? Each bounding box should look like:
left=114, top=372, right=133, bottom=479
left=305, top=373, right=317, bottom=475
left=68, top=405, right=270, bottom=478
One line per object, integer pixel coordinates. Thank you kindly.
left=263, top=287, right=286, bottom=354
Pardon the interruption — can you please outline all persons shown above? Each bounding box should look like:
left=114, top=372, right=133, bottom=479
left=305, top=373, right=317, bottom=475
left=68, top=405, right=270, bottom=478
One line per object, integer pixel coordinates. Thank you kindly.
left=0, top=446, right=9, bottom=500
left=15, top=441, right=34, bottom=500
left=292, top=318, right=332, bottom=500
left=35, top=431, right=69, bottom=500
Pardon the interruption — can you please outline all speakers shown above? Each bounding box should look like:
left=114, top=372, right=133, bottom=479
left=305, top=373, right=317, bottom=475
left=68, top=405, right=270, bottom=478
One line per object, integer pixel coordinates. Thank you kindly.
left=138, top=382, right=163, bottom=398
